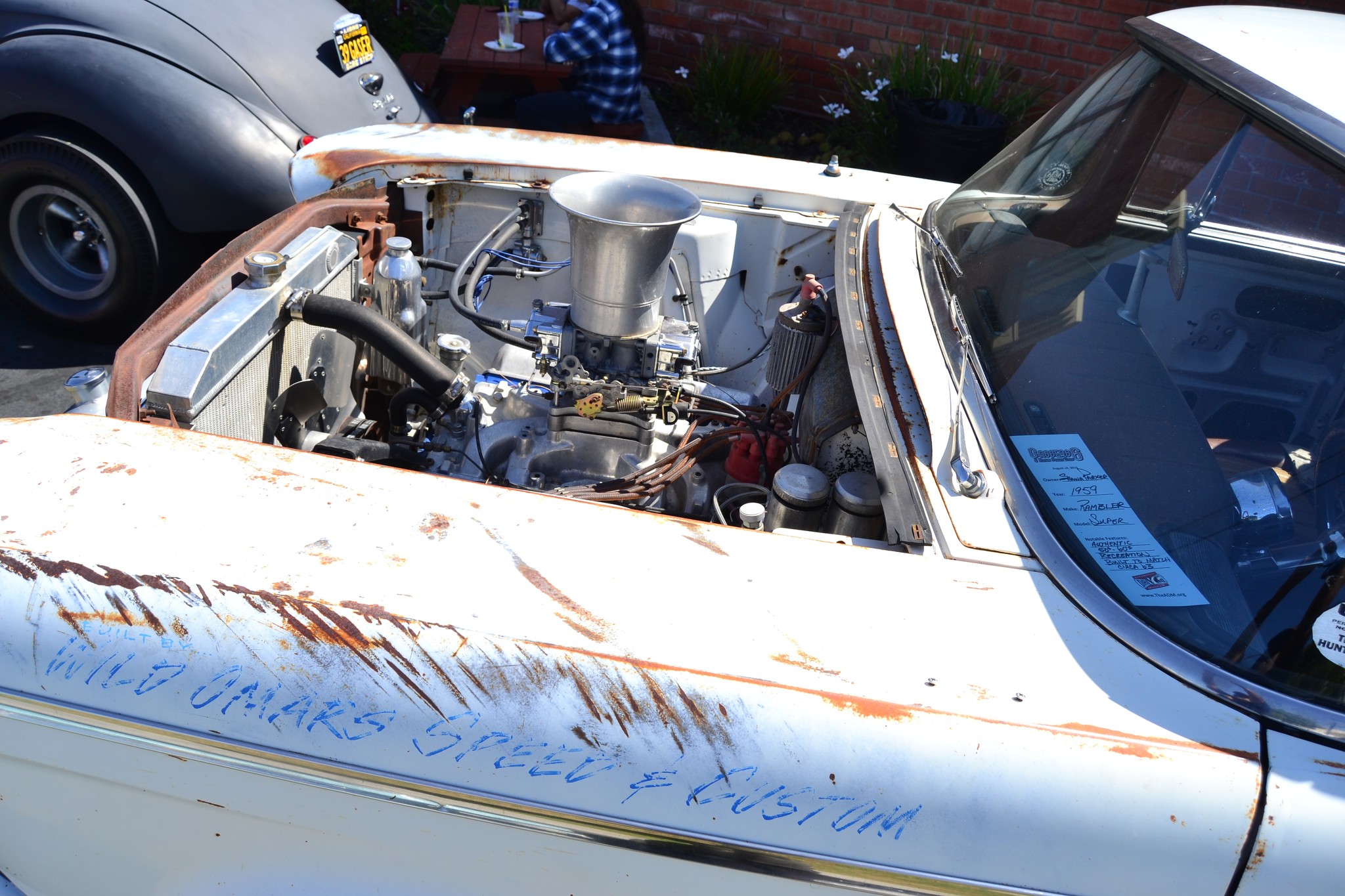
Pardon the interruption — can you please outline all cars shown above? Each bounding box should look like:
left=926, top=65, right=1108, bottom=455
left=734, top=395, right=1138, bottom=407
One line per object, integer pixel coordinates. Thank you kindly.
left=0, top=0, right=1345, bottom=893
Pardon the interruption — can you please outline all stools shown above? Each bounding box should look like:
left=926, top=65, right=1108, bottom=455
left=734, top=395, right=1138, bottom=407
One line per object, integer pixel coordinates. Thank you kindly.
left=592, top=120, right=643, bottom=138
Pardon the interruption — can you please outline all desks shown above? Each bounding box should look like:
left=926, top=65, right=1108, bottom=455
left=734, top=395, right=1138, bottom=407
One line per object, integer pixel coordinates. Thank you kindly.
left=436, top=4, right=572, bottom=115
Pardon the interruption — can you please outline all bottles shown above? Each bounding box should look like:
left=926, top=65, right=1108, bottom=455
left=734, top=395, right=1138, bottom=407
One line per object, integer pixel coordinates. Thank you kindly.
left=508, top=0, right=520, bottom=24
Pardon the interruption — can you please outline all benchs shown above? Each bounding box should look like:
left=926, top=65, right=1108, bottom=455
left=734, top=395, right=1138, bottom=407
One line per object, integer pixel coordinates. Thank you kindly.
left=399, top=52, right=440, bottom=94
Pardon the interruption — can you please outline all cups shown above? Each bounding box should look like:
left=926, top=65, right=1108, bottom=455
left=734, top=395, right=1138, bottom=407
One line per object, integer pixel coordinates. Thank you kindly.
left=497, top=12, right=516, bottom=48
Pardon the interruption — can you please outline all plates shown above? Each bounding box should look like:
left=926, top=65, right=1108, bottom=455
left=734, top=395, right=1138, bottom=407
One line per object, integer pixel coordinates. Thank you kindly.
left=516, top=9, right=544, bottom=21
left=484, top=41, right=525, bottom=53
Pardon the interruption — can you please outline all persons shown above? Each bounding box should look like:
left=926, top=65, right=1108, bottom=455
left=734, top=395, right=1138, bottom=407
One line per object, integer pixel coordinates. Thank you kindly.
left=517, top=0, right=645, bottom=133
left=540, top=0, right=591, bottom=25
